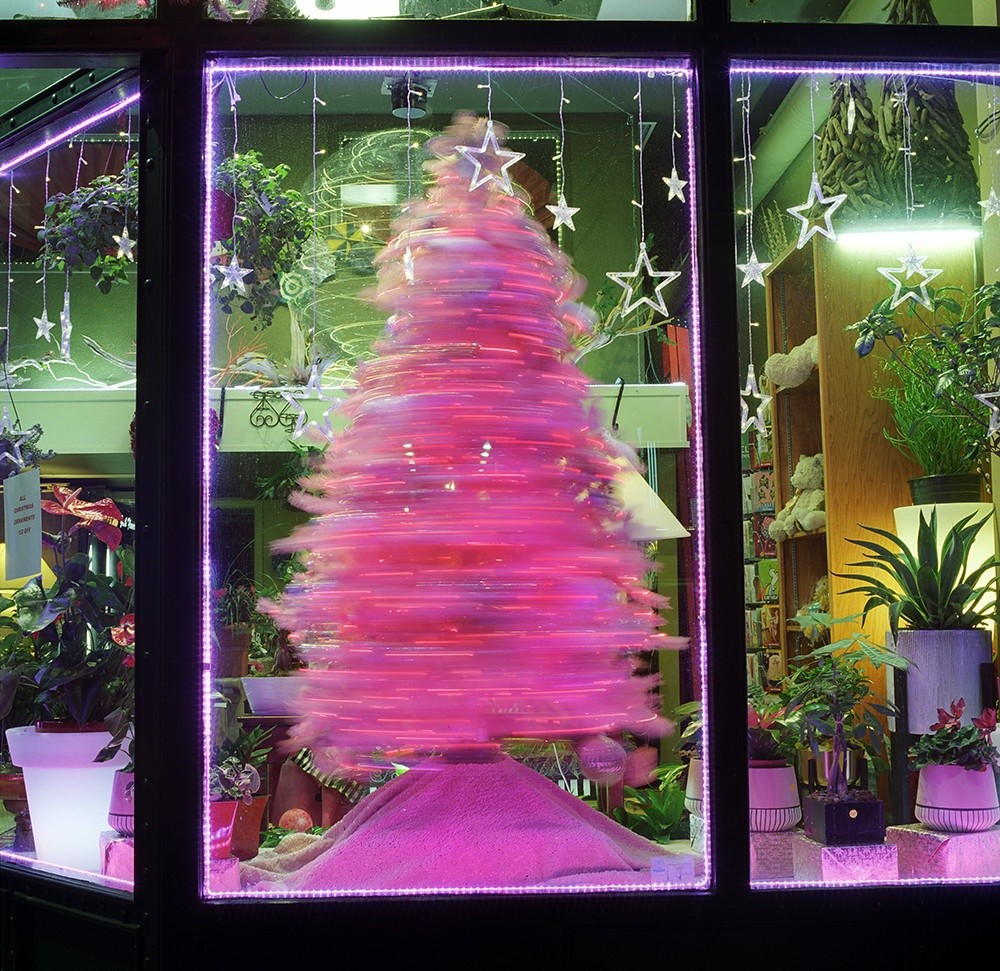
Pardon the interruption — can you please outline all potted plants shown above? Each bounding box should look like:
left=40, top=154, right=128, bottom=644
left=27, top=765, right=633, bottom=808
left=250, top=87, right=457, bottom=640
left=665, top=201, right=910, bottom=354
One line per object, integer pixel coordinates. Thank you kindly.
left=764, top=608, right=923, bottom=847
left=831, top=500, right=1000, bottom=740
left=150, top=573, right=328, bottom=878
left=852, top=273, right=1000, bottom=505
left=35, top=136, right=316, bottom=338
left=676, top=682, right=809, bottom=833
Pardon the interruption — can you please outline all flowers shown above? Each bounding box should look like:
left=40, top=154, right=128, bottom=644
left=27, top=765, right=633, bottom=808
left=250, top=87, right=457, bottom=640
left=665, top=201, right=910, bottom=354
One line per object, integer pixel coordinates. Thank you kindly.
left=905, top=697, right=1000, bottom=775
left=0, top=472, right=141, bottom=727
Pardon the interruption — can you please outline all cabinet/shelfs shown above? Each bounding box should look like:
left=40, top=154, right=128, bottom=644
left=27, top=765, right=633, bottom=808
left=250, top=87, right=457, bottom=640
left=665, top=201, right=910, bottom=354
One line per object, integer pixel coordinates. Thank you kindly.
left=761, top=218, right=984, bottom=830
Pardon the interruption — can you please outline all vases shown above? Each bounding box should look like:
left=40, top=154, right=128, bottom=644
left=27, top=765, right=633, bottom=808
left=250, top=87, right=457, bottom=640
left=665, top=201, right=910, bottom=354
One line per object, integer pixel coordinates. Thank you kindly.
left=1, top=718, right=139, bottom=880
left=912, top=761, right=1000, bottom=832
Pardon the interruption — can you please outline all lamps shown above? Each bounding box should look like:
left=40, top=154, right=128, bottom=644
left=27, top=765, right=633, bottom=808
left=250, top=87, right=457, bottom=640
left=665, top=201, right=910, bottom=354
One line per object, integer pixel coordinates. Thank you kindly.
left=377, top=73, right=443, bottom=121
